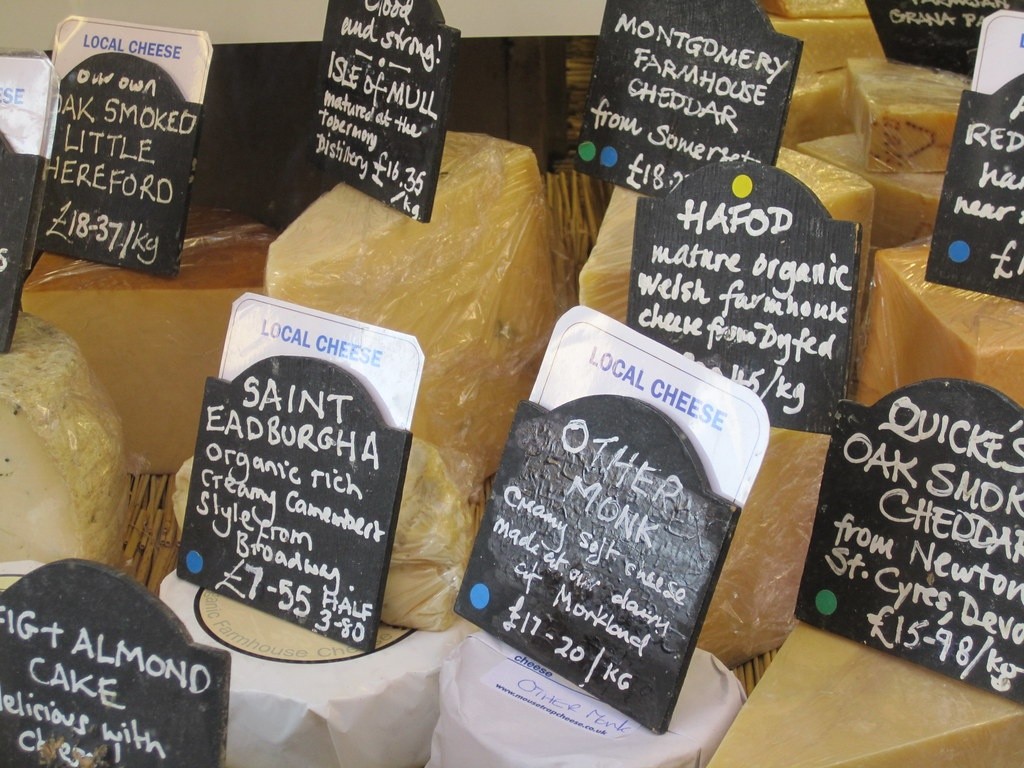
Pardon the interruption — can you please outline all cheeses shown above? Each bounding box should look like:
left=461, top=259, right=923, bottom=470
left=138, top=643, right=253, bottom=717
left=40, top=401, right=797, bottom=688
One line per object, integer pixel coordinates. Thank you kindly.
left=0, top=0, right=1024, bottom=768
